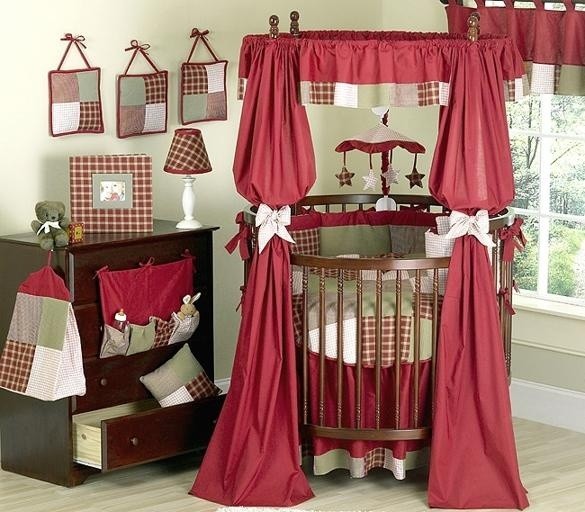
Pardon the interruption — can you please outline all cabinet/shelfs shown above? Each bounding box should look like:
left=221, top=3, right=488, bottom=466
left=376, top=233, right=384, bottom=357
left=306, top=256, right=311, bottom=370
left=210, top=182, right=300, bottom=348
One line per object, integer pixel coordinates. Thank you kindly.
left=1, top=219, right=229, bottom=489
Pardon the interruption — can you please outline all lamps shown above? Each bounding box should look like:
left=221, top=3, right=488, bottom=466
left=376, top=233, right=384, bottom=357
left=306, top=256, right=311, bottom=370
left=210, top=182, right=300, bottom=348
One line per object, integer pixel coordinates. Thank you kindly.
left=162, top=128, right=213, bottom=230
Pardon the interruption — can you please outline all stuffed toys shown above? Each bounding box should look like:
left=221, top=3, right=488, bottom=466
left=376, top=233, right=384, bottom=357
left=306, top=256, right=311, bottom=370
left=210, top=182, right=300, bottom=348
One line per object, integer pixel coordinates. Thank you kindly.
left=177, top=291, right=202, bottom=319
left=30, top=199, right=70, bottom=251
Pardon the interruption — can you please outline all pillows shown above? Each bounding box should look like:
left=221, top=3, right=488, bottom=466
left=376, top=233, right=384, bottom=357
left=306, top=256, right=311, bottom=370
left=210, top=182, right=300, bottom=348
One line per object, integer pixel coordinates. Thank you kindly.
left=139, top=343, right=222, bottom=408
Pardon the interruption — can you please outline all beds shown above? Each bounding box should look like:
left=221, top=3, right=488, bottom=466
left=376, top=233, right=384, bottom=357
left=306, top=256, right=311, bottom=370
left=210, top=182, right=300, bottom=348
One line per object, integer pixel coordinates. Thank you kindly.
left=238, top=193, right=517, bottom=501
